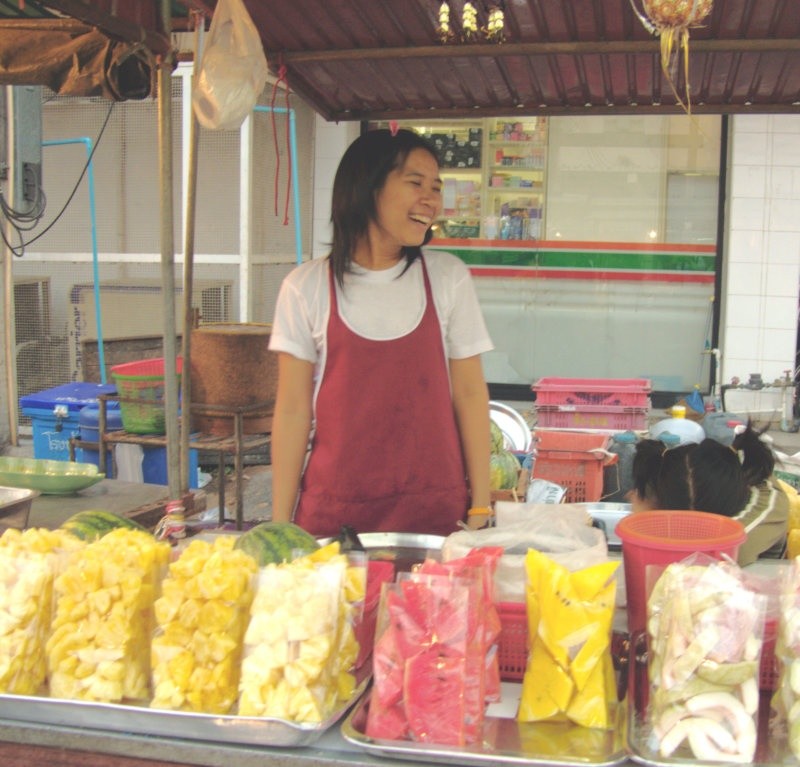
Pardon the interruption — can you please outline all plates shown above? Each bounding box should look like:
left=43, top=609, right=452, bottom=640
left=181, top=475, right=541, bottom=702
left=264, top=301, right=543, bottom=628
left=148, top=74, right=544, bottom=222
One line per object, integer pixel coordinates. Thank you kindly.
left=0, top=457, right=107, bottom=495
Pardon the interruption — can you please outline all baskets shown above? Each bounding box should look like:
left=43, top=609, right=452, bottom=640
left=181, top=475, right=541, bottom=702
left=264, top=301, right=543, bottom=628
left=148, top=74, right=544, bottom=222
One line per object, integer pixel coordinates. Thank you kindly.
left=614, top=509, right=747, bottom=641
left=495, top=600, right=527, bottom=681
left=113, top=355, right=184, bottom=435
left=724, top=575, right=800, bottom=691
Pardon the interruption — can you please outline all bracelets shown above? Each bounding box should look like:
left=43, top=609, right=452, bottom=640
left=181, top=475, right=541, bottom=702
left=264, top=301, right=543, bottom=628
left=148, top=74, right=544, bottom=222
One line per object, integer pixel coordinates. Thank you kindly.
left=467, top=506, right=495, bottom=517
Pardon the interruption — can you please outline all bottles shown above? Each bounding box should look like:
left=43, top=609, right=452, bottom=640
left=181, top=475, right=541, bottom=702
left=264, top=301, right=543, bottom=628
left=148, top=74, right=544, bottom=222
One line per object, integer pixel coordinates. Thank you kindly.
left=650, top=405, right=706, bottom=447
left=605, top=433, right=639, bottom=502
left=658, top=432, right=680, bottom=455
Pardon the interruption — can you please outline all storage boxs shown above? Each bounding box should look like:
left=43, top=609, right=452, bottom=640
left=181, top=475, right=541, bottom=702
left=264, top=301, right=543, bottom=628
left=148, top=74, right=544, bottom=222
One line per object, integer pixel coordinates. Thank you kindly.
left=488, top=376, right=652, bottom=504
left=19, top=380, right=184, bottom=461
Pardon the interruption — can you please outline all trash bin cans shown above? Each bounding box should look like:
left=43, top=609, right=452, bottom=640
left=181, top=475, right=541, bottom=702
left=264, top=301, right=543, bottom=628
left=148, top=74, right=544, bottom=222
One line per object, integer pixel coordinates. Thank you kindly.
left=19, top=381, right=200, bottom=490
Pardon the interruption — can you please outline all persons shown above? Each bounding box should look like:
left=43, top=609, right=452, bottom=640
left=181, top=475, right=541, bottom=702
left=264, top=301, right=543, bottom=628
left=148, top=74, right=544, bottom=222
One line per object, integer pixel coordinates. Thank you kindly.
left=631, top=415, right=800, bottom=569
left=267, top=129, right=495, bottom=540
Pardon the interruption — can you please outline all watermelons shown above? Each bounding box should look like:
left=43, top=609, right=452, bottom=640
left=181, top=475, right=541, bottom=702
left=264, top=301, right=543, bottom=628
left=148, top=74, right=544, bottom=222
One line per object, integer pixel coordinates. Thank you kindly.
left=366, top=545, right=506, bottom=746
left=489, top=420, right=519, bottom=490
left=59, top=508, right=154, bottom=542
left=232, top=521, right=323, bottom=567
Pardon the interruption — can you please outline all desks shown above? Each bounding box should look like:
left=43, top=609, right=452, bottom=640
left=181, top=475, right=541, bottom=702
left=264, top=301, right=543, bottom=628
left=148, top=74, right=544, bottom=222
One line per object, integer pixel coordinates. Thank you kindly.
left=0, top=478, right=210, bottom=539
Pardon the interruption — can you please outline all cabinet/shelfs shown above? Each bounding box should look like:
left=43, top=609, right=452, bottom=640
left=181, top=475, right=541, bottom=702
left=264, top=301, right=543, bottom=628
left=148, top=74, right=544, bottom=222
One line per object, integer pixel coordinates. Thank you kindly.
left=97, top=391, right=275, bottom=531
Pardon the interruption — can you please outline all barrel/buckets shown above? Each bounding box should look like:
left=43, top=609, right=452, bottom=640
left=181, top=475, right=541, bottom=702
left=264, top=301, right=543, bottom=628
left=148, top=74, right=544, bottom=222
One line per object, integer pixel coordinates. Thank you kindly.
left=614, top=511, right=748, bottom=640
